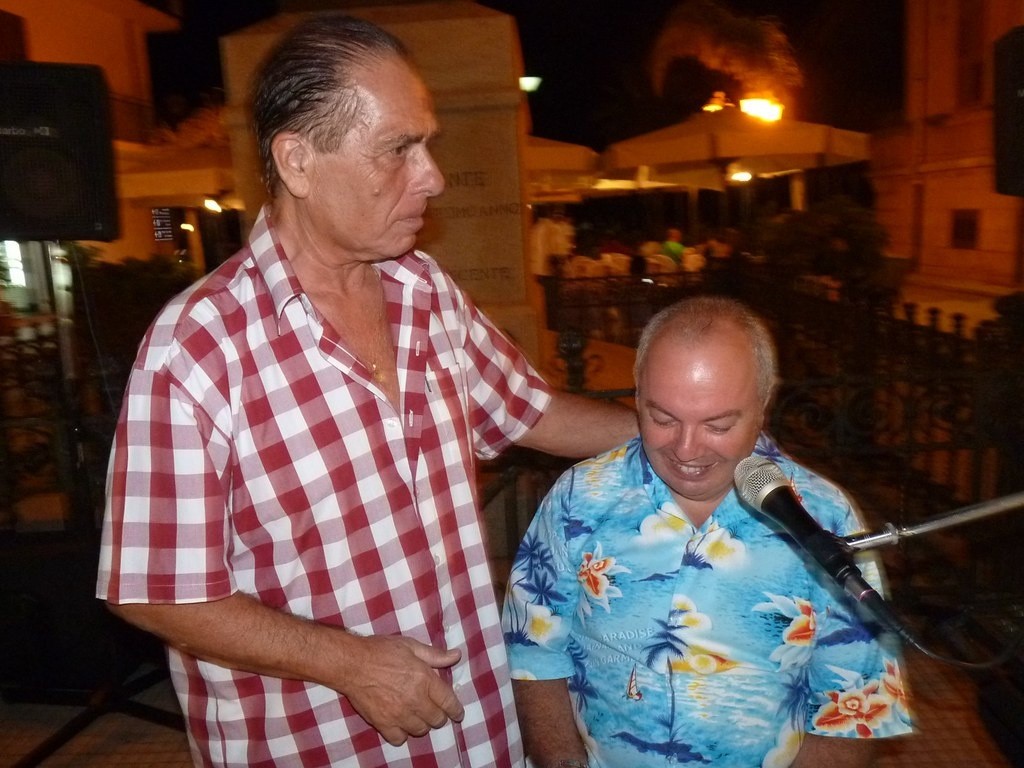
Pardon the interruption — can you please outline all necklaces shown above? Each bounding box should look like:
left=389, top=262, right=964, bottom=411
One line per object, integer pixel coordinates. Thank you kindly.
left=373, top=270, right=388, bottom=384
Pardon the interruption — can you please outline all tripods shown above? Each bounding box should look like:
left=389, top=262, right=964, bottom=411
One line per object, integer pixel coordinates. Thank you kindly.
left=11, top=241, right=185, bottom=768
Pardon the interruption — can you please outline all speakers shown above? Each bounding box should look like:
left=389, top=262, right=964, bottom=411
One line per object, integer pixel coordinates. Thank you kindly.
left=994, top=24, right=1024, bottom=195
left=0, top=59, right=121, bottom=243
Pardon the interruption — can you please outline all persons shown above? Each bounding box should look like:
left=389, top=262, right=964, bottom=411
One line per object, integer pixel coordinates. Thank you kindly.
left=95, top=14, right=642, bottom=768
left=501, top=298, right=913, bottom=768
left=532, top=202, right=576, bottom=326
left=663, top=227, right=738, bottom=264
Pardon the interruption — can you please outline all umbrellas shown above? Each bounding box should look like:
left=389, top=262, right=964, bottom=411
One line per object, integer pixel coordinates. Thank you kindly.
left=526, top=99, right=869, bottom=206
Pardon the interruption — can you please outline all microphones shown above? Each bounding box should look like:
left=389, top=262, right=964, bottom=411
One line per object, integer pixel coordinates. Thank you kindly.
left=734, top=456, right=917, bottom=645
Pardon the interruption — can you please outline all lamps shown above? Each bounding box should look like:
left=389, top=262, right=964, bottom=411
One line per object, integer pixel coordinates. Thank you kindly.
left=701, top=91, right=735, bottom=113
left=738, top=91, right=785, bottom=123
left=203, top=189, right=227, bottom=213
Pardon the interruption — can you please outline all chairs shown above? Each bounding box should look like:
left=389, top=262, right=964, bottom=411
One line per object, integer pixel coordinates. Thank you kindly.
left=565, top=240, right=732, bottom=318
